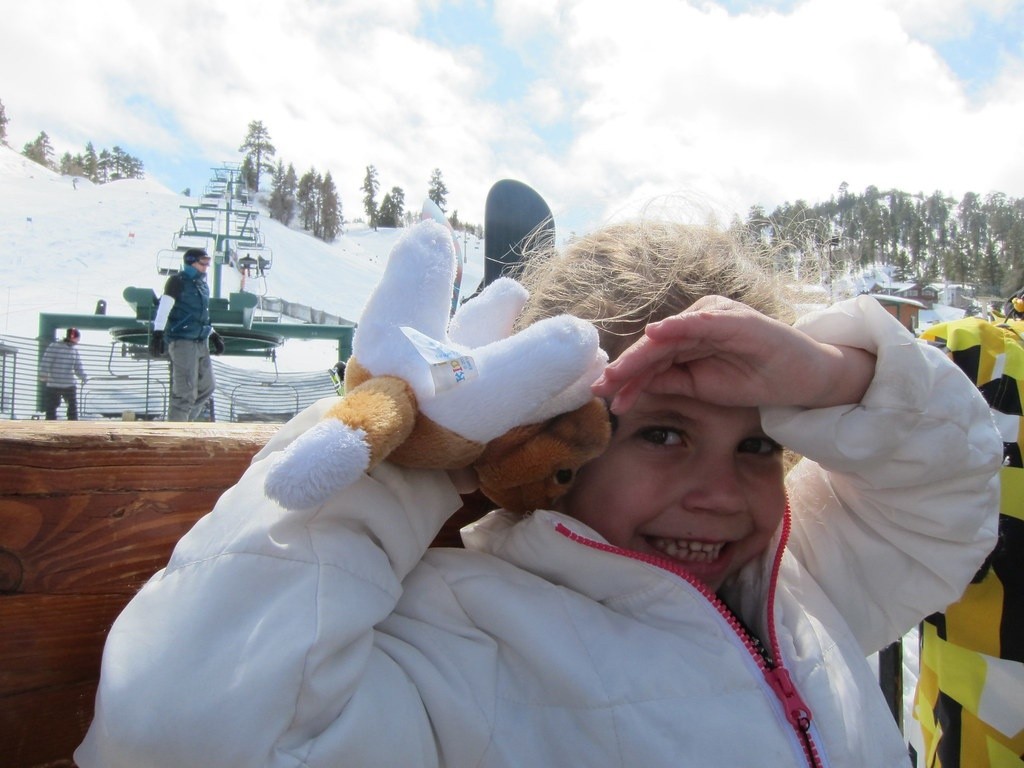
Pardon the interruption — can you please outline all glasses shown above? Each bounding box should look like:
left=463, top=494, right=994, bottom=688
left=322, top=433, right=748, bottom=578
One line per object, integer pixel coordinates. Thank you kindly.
left=199, top=260, right=207, bottom=265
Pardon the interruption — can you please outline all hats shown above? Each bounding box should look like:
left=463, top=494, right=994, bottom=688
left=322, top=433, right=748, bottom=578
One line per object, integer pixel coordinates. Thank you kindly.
left=185, top=249, right=209, bottom=266
left=67, top=328, right=78, bottom=339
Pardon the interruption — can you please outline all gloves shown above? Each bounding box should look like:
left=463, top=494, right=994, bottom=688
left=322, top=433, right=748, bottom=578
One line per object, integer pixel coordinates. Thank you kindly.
left=210, top=332, right=224, bottom=354
left=149, top=330, right=165, bottom=358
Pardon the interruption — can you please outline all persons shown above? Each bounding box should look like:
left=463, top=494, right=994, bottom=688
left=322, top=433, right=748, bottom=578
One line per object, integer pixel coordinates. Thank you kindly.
left=910, top=289, right=1024, bottom=768
left=239, top=253, right=266, bottom=275
left=39, top=328, right=86, bottom=420
left=148, top=247, right=224, bottom=421
left=74, top=221, right=1004, bottom=768
